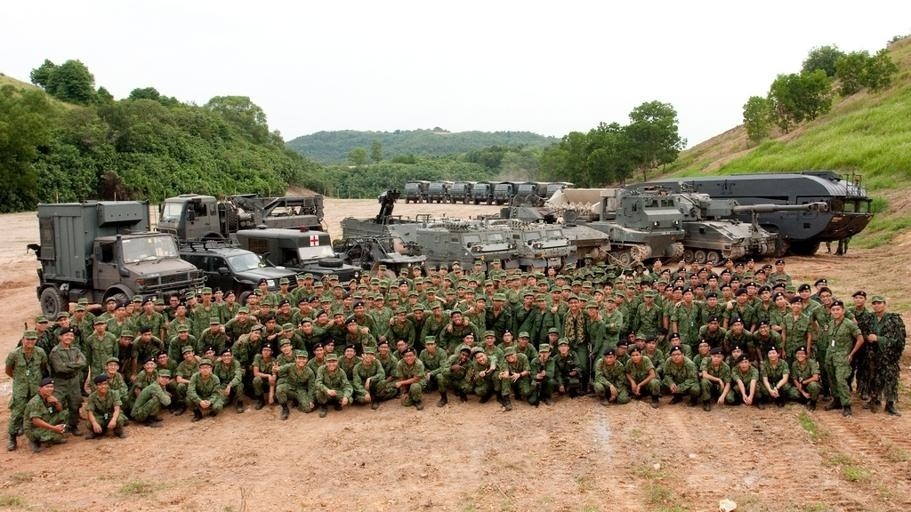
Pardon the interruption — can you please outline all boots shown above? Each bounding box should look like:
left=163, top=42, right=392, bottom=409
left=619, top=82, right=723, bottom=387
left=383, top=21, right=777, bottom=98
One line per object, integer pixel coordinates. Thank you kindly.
left=501, top=394, right=512, bottom=412
left=650, top=395, right=660, bottom=409
left=436, top=391, right=448, bottom=409
left=253, top=394, right=265, bottom=410
left=279, top=401, right=289, bottom=421
left=318, top=402, right=328, bottom=419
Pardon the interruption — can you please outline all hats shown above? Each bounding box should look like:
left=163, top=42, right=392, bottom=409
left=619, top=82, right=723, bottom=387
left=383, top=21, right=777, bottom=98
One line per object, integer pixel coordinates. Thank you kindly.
left=767, top=345, right=778, bottom=353
left=93, top=317, right=106, bottom=326
left=871, top=295, right=885, bottom=304
left=54, top=311, right=69, bottom=319
left=139, top=326, right=152, bottom=334
left=142, top=345, right=233, bottom=379
left=175, top=324, right=189, bottom=334
left=104, top=294, right=165, bottom=311
left=105, top=356, right=120, bottom=365
left=850, top=290, right=867, bottom=299
left=120, top=329, right=134, bottom=338
left=34, top=315, right=49, bottom=323
left=22, top=330, right=38, bottom=341
left=174, top=287, right=234, bottom=310
left=208, top=316, right=219, bottom=325
left=37, top=377, right=55, bottom=388
left=73, top=297, right=88, bottom=312
left=238, top=258, right=788, bottom=332
left=58, top=326, right=74, bottom=336
left=262, top=331, right=754, bottom=362
left=795, top=345, right=807, bottom=353
left=785, top=278, right=845, bottom=310
left=93, top=375, right=108, bottom=385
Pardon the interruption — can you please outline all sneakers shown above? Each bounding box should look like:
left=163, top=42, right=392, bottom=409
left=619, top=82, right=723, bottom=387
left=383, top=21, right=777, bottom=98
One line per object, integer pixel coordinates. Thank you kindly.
left=190, top=409, right=217, bottom=423
left=68, top=425, right=100, bottom=440
left=600, top=397, right=610, bottom=406
left=145, top=414, right=163, bottom=429
left=370, top=398, right=380, bottom=410
left=701, top=398, right=711, bottom=412
left=5, top=435, right=17, bottom=452
left=808, top=399, right=817, bottom=412
left=112, top=429, right=128, bottom=439
left=413, top=400, right=424, bottom=411
left=234, top=400, right=244, bottom=414
left=168, top=406, right=186, bottom=417
left=859, top=389, right=903, bottom=417
left=822, top=397, right=852, bottom=417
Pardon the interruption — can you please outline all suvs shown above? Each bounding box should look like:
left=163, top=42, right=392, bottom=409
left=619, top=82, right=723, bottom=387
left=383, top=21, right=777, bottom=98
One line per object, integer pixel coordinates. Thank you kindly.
left=175, top=234, right=299, bottom=300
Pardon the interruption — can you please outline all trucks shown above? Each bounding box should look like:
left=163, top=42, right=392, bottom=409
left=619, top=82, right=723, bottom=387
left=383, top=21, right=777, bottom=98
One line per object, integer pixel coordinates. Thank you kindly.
left=402, top=176, right=574, bottom=206
left=28, top=195, right=211, bottom=319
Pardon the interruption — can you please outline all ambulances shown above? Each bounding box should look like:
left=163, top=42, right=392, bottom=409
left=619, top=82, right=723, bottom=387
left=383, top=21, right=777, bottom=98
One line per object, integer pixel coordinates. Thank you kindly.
left=233, top=221, right=361, bottom=290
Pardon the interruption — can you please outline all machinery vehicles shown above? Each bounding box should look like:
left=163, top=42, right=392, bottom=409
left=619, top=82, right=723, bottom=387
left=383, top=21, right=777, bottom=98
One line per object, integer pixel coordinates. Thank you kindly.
left=155, top=189, right=325, bottom=248
